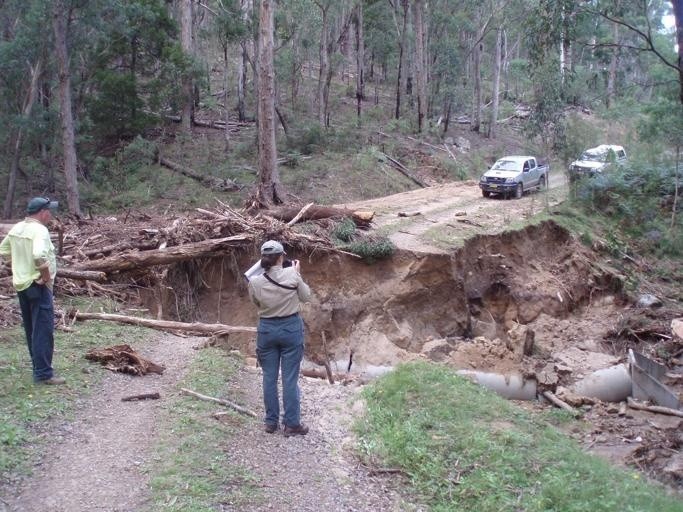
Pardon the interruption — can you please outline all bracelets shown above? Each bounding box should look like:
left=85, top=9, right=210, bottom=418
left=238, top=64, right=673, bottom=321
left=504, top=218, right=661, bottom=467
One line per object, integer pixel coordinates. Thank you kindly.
left=40, top=277, right=47, bottom=283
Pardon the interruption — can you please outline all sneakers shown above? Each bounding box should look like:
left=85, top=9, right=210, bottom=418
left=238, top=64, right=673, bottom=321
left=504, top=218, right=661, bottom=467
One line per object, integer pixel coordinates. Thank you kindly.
left=264, top=423, right=280, bottom=433
left=283, top=424, right=309, bottom=437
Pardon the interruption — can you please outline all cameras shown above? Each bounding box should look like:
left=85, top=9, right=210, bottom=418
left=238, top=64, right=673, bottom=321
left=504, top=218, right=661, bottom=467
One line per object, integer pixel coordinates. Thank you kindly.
left=283, top=259, right=296, bottom=268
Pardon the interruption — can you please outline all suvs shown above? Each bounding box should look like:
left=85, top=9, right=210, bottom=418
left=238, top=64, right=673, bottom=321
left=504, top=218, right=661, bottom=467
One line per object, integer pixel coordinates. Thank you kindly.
left=567, top=144, right=628, bottom=180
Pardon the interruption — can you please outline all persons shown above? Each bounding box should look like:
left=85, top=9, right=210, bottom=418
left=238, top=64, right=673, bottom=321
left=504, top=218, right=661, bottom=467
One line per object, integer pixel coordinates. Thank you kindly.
left=0, top=197, right=66, bottom=386
left=247, top=240, right=311, bottom=436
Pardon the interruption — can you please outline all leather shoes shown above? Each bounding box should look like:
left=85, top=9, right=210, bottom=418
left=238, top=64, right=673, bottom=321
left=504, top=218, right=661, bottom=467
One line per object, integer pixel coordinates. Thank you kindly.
left=35, top=375, right=66, bottom=386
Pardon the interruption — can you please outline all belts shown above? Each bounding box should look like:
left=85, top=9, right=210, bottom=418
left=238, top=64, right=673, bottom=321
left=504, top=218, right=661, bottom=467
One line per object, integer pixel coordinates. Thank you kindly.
left=259, top=311, right=298, bottom=321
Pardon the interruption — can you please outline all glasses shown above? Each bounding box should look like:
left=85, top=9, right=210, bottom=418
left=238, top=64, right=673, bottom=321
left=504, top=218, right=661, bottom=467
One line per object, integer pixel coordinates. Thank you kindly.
left=39, top=197, right=50, bottom=211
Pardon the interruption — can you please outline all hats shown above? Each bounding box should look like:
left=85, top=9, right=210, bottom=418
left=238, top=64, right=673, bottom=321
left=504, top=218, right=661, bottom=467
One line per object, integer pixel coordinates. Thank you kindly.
left=25, top=196, right=61, bottom=213
left=260, top=239, right=287, bottom=256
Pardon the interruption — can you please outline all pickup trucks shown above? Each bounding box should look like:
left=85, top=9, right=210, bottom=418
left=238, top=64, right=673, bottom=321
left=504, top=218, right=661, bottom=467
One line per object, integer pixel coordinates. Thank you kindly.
left=479, top=156, right=550, bottom=198
